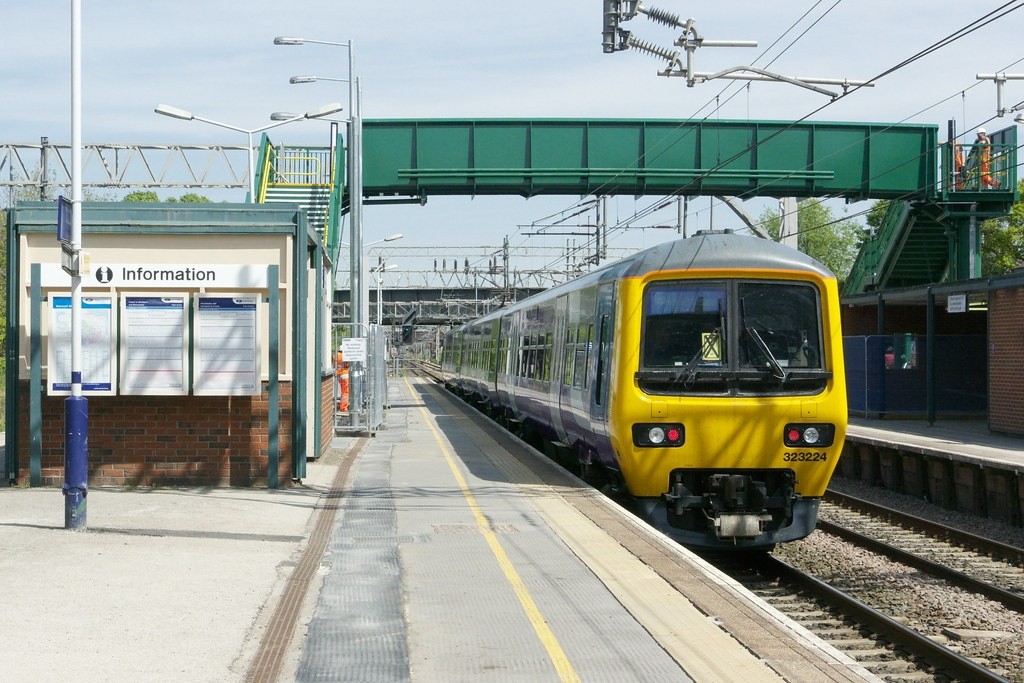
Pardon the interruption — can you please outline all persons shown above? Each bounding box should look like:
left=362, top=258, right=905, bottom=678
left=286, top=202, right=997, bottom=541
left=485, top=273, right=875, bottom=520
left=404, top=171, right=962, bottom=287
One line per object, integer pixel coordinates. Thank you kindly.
left=333, top=344, right=349, bottom=413
left=954, top=140, right=965, bottom=190
left=974, top=128, right=1001, bottom=190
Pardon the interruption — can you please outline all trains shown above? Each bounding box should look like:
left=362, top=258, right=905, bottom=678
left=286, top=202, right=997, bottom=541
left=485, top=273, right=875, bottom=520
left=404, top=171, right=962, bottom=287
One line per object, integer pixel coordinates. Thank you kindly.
left=441, top=225, right=850, bottom=555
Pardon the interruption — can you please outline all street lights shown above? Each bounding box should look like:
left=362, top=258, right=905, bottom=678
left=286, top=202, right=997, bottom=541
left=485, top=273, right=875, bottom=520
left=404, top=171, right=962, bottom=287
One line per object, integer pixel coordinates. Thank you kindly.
left=268, top=33, right=403, bottom=432
left=153, top=99, right=344, bottom=204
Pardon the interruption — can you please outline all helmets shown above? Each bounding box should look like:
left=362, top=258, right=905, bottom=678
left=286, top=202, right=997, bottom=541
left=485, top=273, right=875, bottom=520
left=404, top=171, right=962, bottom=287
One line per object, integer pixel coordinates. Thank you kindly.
left=337, top=345, right=343, bottom=352
